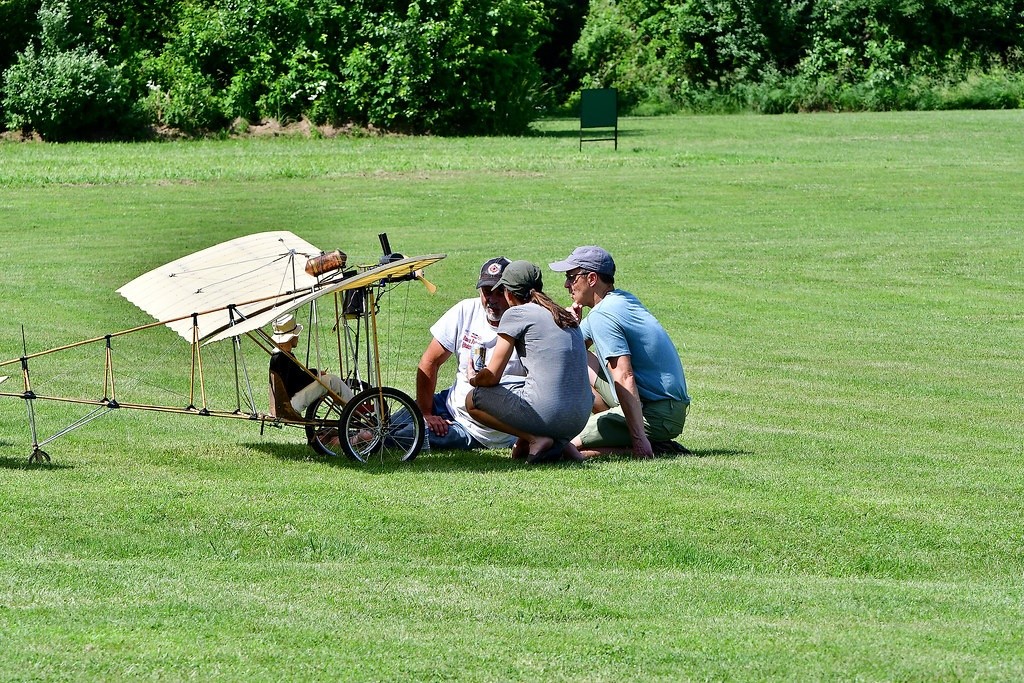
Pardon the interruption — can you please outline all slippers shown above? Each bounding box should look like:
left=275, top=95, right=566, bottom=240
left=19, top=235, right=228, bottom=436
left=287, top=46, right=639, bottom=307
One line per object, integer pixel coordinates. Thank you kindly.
left=527, top=448, right=561, bottom=463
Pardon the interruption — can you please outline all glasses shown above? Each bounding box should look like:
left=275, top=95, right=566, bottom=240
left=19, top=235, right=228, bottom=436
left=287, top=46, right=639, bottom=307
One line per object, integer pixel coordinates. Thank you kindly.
left=566, top=272, right=590, bottom=283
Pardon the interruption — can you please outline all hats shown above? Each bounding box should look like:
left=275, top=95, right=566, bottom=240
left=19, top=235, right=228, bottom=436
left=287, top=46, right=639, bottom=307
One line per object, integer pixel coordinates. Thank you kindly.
left=476, top=256, right=513, bottom=289
left=491, top=260, right=543, bottom=296
left=549, top=245, right=616, bottom=276
left=270, top=314, right=303, bottom=344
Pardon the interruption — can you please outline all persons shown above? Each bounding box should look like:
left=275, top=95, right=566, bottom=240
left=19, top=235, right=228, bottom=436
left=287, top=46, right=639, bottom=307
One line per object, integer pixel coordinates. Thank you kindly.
left=267, top=315, right=375, bottom=415
left=549, top=246, right=689, bottom=458
left=310, top=256, right=527, bottom=452
left=464, top=261, right=596, bottom=460
